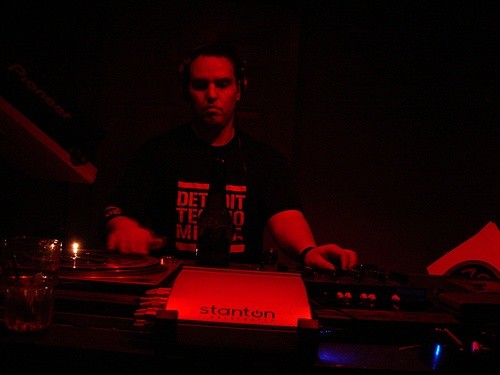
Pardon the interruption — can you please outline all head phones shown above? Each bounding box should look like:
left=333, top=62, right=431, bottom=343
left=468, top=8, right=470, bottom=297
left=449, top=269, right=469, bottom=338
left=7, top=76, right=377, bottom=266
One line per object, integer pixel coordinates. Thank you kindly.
left=170, top=43, right=255, bottom=97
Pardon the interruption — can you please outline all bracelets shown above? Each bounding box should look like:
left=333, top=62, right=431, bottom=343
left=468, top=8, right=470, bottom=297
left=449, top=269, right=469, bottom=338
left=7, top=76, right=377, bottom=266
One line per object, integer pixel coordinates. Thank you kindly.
left=298, top=245, right=315, bottom=265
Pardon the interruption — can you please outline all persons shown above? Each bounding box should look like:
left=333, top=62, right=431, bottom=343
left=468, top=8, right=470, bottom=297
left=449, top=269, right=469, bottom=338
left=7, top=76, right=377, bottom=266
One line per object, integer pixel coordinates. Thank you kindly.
left=101, top=42, right=357, bottom=272
